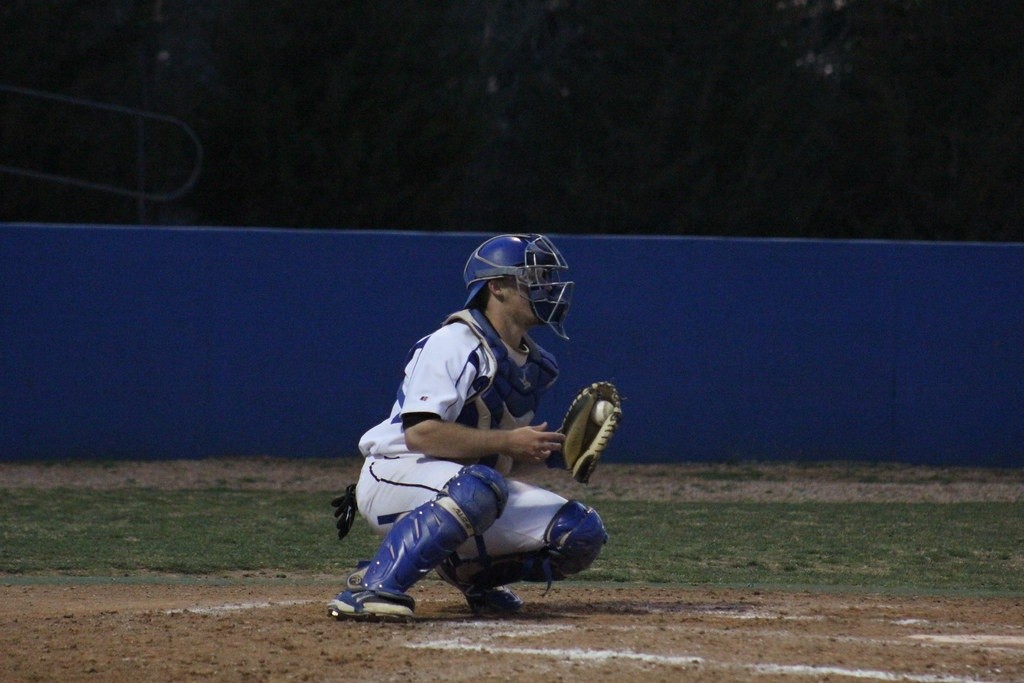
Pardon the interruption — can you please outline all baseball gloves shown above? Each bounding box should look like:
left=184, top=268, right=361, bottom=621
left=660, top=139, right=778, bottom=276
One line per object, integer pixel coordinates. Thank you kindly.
left=553, top=380, right=623, bottom=484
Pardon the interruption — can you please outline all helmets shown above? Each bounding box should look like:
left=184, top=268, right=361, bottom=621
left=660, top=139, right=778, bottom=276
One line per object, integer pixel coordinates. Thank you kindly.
left=462, top=234, right=553, bottom=310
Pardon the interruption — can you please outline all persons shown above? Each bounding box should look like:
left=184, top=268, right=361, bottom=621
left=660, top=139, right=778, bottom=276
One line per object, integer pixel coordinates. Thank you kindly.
left=326, top=235, right=621, bottom=616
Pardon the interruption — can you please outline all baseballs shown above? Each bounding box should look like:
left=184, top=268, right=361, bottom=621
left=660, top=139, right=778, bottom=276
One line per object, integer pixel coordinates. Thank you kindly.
left=591, top=400, right=614, bottom=427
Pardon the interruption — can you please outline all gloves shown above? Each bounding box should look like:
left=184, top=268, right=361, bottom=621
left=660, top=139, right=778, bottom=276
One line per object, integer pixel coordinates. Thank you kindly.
left=332, top=485, right=360, bottom=539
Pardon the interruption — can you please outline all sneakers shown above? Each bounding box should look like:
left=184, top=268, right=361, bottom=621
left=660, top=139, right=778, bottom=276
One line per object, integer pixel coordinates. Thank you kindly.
left=438, top=557, right=525, bottom=617
left=327, top=583, right=414, bottom=619
